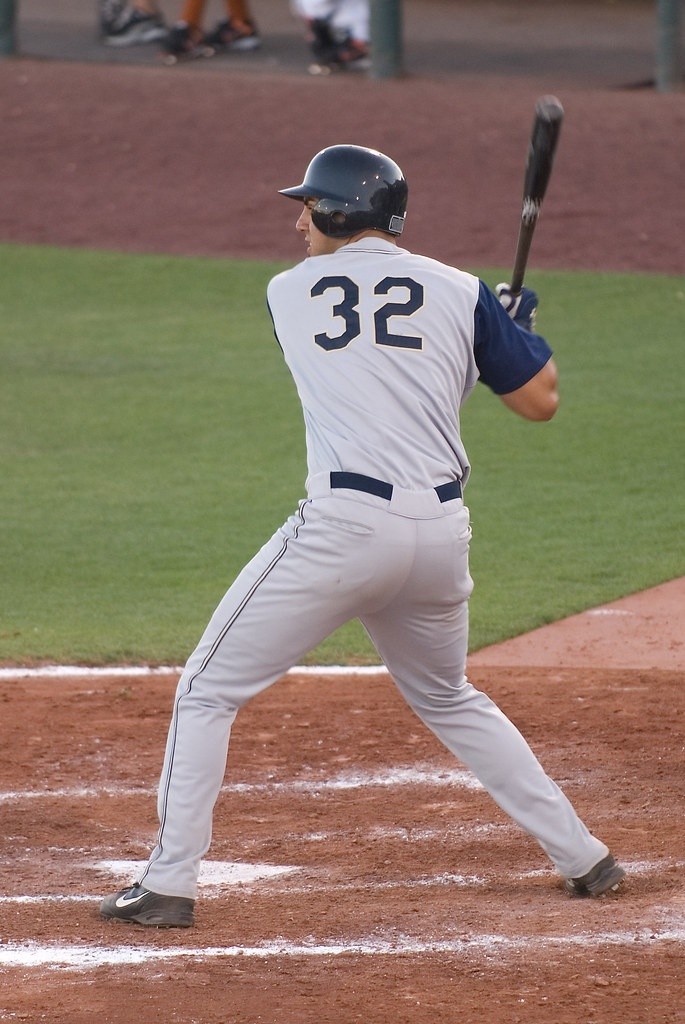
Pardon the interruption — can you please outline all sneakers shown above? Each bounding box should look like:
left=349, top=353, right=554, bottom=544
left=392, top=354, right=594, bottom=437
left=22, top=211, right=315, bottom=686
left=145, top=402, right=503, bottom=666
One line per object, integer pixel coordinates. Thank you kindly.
left=100, top=880, right=195, bottom=927
left=103, top=7, right=167, bottom=46
left=204, top=15, right=258, bottom=48
left=316, top=37, right=373, bottom=72
left=165, top=27, right=211, bottom=61
left=565, top=852, right=626, bottom=898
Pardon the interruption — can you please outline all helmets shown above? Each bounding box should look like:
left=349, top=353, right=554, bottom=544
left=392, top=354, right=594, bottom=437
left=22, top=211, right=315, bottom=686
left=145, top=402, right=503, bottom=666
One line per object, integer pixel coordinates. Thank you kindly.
left=278, top=145, right=407, bottom=236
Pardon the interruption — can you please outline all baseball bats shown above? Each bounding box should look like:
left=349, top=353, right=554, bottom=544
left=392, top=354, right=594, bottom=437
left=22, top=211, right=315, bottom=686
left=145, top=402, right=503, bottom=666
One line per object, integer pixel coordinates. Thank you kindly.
left=509, top=93, right=565, bottom=295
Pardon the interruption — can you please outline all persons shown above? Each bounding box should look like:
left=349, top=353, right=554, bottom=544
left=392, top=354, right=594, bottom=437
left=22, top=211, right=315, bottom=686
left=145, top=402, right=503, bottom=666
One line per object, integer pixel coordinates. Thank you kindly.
left=0, top=0, right=18, bottom=54
left=164, top=0, right=259, bottom=63
left=296, top=0, right=369, bottom=72
left=102, top=144, right=626, bottom=927
left=100, top=0, right=169, bottom=44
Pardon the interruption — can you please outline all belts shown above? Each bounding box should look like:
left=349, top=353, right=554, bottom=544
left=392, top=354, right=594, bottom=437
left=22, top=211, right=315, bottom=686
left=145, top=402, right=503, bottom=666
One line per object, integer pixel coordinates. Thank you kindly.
left=331, top=472, right=462, bottom=503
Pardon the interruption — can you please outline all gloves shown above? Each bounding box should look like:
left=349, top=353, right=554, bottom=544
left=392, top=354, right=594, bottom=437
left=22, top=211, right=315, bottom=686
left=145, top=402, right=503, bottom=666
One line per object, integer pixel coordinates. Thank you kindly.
left=494, top=281, right=539, bottom=331
